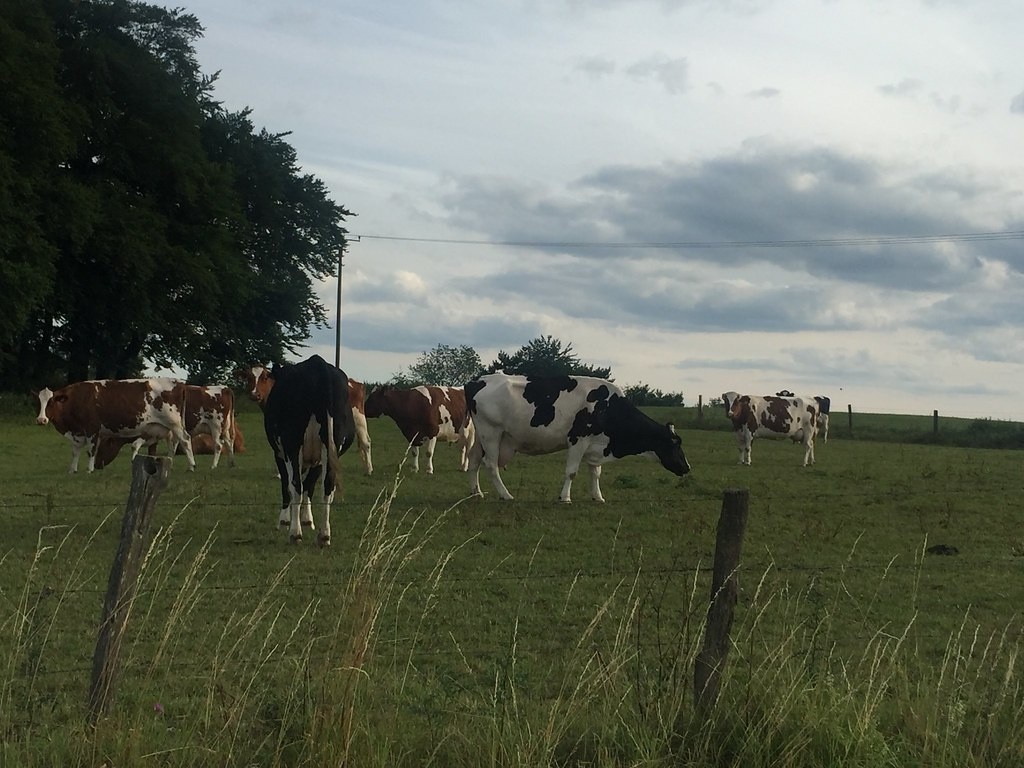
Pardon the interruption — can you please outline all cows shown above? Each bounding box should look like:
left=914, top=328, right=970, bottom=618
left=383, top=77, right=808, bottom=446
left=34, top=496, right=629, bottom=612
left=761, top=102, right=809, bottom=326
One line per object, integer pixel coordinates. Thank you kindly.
left=246, top=354, right=374, bottom=546
left=462, top=372, right=693, bottom=504
left=722, top=390, right=831, bottom=467
left=363, top=385, right=476, bottom=474
left=30, top=378, right=245, bottom=477
